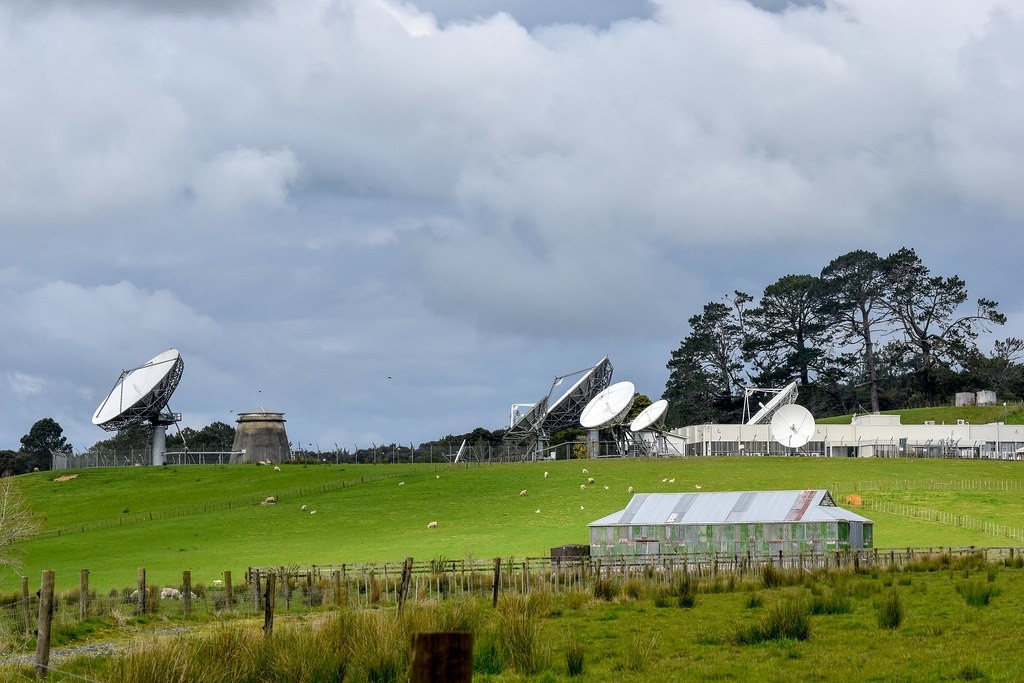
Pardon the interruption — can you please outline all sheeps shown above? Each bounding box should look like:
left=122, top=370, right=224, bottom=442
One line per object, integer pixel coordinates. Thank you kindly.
left=426, top=521, right=437, bottom=529
left=544, top=472, right=548, bottom=478
left=588, top=478, right=595, bottom=484
left=582, top=468, right=588, bottom=473
left=580, top=485, right=586, bottom=490
left=311, top=510, right=316, bottom=514
left=436, top=474, right=440, bottom=479
left=301, top=505, right=307, bottom=510
left=628, top=486, right=633, bottom=493
left=265, top=497, right=274, bottom=501
left=399, top=481, right=405, bottom=486
left=520, top=490, right=528, bottom=497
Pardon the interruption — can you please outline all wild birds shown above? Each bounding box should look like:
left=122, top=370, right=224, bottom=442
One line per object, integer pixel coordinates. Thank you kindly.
left=669, top=479, right=676, bottom=482
left=580, top=505, right=584, bottom=510
left=604, top=486, right=609, bottom=490
left=535, top=509, right=540, bottom=514
left=663, top=479, right=668, bottom=483
left=695, top=485, right=701, bottom=489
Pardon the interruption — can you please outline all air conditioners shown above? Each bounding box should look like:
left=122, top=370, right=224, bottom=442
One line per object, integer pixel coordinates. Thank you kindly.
left=956, top=420, right=965, bottom=425
left=812, top=453, right=819, bottom=457
left=753, top=453, right=762, bottom=456
left=799, top=453, right=806, bottom=457
left=924, top=420, right=936, bottom=426
